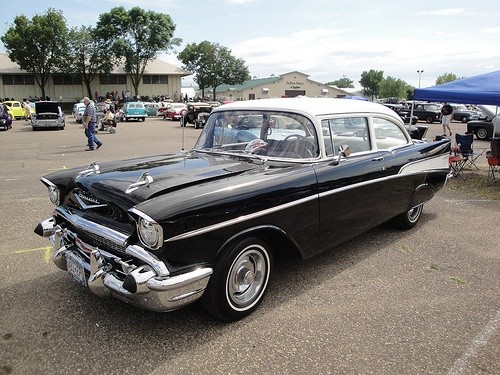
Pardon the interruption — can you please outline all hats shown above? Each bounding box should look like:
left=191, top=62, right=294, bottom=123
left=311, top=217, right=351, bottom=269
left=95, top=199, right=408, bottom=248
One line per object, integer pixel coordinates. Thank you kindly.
left=82, top=97, right=90, bottom=101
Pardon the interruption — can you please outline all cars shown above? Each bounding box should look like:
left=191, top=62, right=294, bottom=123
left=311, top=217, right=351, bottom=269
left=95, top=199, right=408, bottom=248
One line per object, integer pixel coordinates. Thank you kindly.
left=30, top=101, right=66, bottom=130
left=21, top=102, right=36, bottom=119
left=72, top=99, right=237, bottom=134
left=0, top=102, right=13, bottom=132
left=34, top=97, right=452, bottom=324
left=379, top=96, right=500, bottom=140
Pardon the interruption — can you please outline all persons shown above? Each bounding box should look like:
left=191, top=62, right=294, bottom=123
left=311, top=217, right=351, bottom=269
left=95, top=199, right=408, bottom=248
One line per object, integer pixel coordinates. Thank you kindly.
left=439, top=101, right=453, bottom=136
left=144, top=91, right=179, bottom=103
left=98, top=107, right=113, bottom=131
left=22, top=95, right=50, bottom=108
left=102, top=90, right=129, bottom=122
left=82, top=97, right=102, bottom=151
left=25, top=101, right=33, bottom=121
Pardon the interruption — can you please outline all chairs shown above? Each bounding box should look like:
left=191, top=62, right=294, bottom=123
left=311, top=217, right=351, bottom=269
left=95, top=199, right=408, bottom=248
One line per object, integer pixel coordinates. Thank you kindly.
left=435, top=133, right=500, bottom=187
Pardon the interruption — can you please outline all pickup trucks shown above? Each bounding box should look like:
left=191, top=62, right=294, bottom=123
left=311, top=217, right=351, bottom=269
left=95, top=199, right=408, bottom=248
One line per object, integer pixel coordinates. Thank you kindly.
left=3, top=101, right=28, bottom=121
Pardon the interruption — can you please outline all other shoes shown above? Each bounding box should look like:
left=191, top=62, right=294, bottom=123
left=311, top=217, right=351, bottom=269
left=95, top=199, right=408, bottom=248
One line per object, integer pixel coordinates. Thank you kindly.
left=85, top=148, right=94, bottom=151
left=96, top=143, right=102, bottom=149
left=450, top=133, right=452, bottom=136
left=443, top=134, right=447, bottom=136
left=99, top=129, right=104, bottom=131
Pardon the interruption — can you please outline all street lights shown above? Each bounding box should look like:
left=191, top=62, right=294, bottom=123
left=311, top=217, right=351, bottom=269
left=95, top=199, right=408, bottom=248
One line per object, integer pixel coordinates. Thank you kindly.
left=417, top=70, right=424, bottom=88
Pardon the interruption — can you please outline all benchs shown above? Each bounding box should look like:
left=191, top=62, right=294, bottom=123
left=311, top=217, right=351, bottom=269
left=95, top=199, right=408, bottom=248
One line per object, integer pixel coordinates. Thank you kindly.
left=308, top=136, right=400, bottom=154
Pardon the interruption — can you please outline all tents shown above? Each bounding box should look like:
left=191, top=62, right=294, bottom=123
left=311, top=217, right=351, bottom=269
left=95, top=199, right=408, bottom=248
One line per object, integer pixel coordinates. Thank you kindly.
left=409, top=70, right=500, bottom=134
left=342, top=96, right=368, bottom=101
